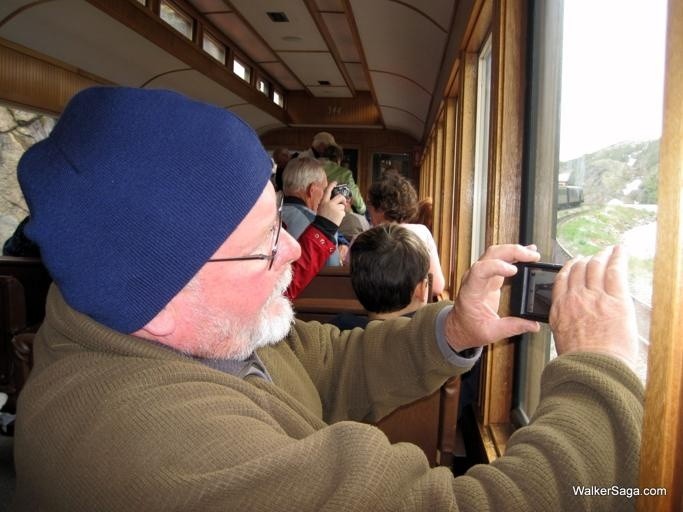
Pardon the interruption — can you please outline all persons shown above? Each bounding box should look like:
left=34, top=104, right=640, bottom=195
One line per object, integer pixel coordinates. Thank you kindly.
left=7, top=85, right=643, bottom=512
left=271, top=132, right=479, bottom=418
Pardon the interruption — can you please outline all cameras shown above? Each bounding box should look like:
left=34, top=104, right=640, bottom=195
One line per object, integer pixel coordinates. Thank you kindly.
left=506, top=261, right=563, bottom=324
left=331, top=183, right=352, bottom=201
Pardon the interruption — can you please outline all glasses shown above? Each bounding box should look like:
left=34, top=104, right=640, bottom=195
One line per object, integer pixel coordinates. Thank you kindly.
left=209, top=185, right=286, bottom=270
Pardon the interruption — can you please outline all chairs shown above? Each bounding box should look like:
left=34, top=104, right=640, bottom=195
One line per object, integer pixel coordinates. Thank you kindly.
left=291, top=269, right=461, bottom=473
left=0, top=252, right=52, bottom=395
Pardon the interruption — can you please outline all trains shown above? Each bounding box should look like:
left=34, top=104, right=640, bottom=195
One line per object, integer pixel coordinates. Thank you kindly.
left=558, top=186, right=584, bottom=209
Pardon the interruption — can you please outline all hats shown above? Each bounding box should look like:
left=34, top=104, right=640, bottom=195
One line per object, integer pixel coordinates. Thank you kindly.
left=16, top=83, right=275, bottom=335
